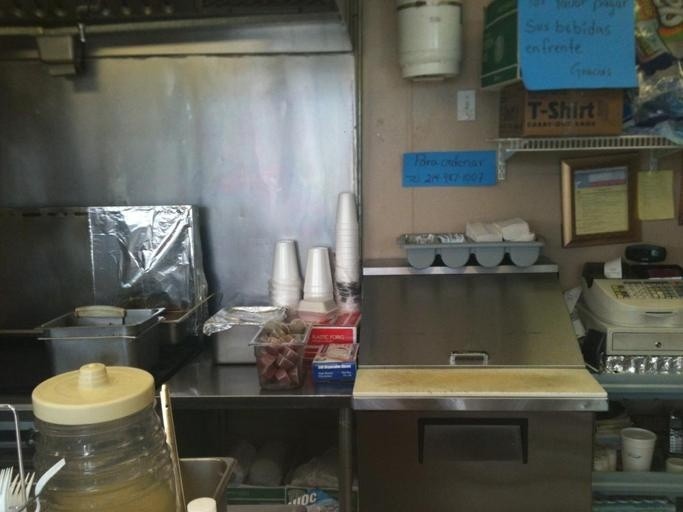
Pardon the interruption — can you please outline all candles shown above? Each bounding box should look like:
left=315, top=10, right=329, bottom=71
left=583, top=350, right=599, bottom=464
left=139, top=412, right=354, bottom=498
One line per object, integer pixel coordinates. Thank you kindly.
left=559, top=153, right=642, bottom=248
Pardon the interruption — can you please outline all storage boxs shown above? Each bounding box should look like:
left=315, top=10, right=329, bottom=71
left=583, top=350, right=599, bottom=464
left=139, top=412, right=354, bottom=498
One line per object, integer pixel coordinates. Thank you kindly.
left=480, top=0, right=634, bottom=92
left=499, top=81, right=624, bottom=137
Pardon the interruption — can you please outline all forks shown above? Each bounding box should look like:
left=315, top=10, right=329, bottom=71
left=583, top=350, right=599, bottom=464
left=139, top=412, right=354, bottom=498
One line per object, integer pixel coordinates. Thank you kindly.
left=0, top=468, right=34, bottom=510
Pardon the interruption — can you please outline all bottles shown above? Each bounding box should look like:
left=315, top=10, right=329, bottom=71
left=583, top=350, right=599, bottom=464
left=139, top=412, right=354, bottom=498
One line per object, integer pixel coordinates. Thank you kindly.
left=592, top=423, right=616, bottom=472
left=267, top=189, right=362, bottom=311
left=620, top=427, right=657, bottom=472
left=28, top=358, right=178, bottom=509
left=665, top=413, right=681, bottom=456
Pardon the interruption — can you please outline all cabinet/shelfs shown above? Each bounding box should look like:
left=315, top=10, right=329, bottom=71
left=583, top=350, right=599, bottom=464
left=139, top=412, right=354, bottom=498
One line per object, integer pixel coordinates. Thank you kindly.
left=154, top=346, right=351, bottom=512
